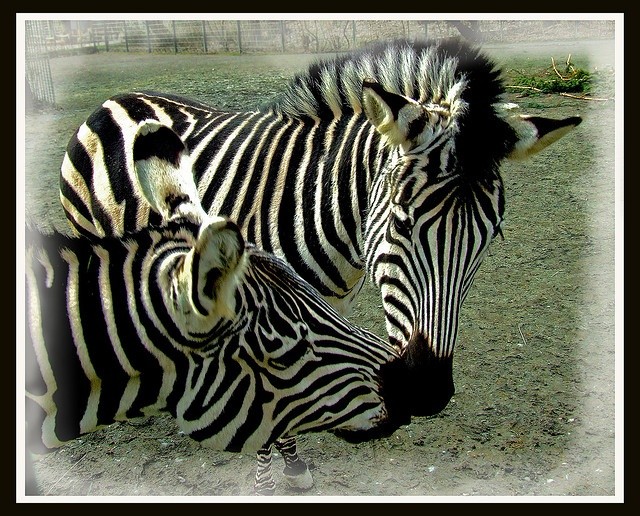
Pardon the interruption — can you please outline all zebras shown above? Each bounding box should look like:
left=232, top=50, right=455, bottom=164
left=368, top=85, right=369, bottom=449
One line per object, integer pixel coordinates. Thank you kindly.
left=23, top=122, right=412, bottom=455
left=59, top=35, right=582, bottom=495
